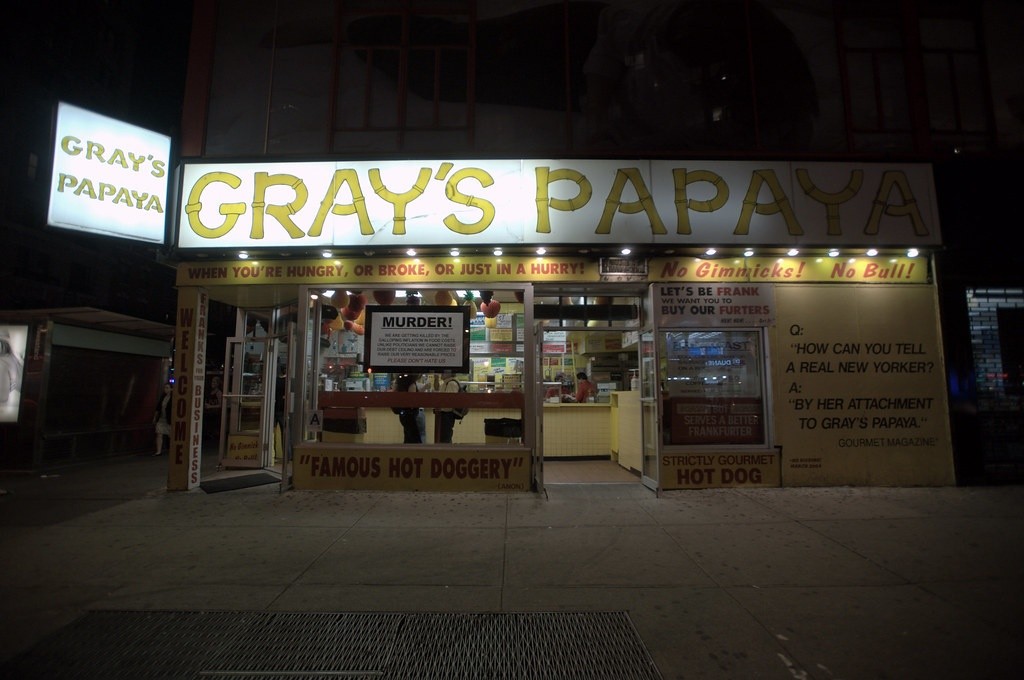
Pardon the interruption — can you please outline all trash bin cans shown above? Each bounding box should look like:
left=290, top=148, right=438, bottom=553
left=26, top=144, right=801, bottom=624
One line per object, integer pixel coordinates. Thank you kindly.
left=320, top=417, right=368, bottom=443
left=482, top=417, right=523, bottom=445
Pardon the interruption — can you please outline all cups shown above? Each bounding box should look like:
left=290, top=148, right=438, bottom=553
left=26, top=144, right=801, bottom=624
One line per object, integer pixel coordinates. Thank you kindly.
left=589, top=396, right=594, bottom=403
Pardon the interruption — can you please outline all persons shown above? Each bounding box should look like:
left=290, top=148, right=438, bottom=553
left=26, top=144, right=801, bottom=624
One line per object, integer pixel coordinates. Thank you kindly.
left=433, top=371, right=461, bottom=444
left=274, top=363, right=294, bottom=463
left=566, top=371, right=598, bottom=403
left=547, top=375, right=570, bottom=402
left=149, top=383, right=173, bottom=457
left=204, top=375, right=222, bottom=469
left=389, top=373, right=431, bottom=444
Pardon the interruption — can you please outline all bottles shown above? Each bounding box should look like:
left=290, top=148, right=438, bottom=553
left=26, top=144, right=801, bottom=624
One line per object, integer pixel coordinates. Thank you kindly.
left=630, top=375, right=639, bottom=391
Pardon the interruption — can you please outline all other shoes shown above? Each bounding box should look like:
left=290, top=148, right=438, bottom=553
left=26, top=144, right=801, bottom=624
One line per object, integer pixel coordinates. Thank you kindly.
left=151, top=453, right=162, bottom=457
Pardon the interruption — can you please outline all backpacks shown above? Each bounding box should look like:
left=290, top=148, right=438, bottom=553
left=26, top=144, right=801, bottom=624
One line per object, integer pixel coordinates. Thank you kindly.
left=443, top=379, right=469, bottom=420
